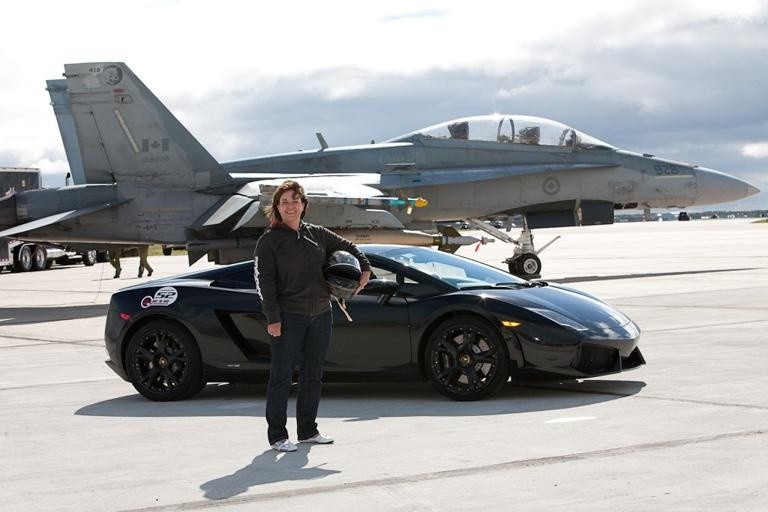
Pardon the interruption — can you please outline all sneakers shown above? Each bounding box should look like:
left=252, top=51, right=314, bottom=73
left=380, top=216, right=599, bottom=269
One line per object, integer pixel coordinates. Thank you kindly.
left=298, top=433, right=334, bottom=444
left=270, top=439, right=298, bottom=452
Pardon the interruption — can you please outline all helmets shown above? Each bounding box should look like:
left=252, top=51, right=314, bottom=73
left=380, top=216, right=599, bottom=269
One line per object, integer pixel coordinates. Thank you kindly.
left=324, top=250, right=362, bottom=299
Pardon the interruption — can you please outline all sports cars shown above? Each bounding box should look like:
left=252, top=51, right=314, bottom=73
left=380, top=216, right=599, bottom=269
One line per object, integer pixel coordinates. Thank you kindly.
left=104, top=244, right=645, bottom=400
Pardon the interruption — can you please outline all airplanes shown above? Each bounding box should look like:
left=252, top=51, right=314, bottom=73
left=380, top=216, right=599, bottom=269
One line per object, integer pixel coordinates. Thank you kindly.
left=1, top=61, right=762, bottom=275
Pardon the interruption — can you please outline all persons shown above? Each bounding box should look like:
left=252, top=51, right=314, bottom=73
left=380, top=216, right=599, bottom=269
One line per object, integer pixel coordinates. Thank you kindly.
left=137, top=246, right=153, bottom=277
left=253, top=180, right=373, bottom=451
left=109, top=247, right=122, bottom=278
